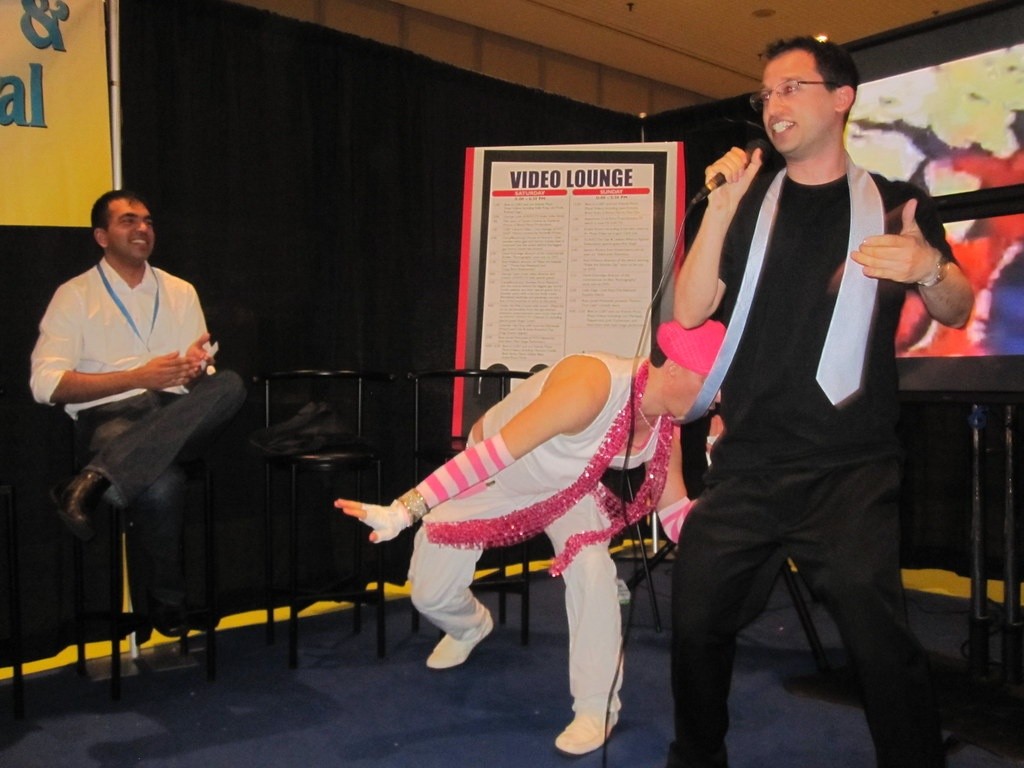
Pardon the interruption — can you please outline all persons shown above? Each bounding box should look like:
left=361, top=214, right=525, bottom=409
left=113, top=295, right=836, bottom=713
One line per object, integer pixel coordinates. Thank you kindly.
left=332, top=319, right=727, bottom=755
left=30, top=190, right=247, bottom=636
left=668, top=34, right=976, bottom=768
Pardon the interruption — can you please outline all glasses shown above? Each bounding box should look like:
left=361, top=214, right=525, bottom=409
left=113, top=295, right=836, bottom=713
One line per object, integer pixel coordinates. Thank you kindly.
left=749, top=79, right=841, bottom=113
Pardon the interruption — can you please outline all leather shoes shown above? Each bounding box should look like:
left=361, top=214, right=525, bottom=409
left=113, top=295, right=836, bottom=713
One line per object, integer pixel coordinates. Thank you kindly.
left=149, top=594, right=189, bottom=635
left=50, top=470, right=112, bottom=539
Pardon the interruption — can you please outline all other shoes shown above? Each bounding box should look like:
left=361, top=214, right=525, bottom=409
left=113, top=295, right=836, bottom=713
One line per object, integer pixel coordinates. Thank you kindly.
left=555, top=713, right=613, bottom=756
left=427, top=618, right=493, bottom=668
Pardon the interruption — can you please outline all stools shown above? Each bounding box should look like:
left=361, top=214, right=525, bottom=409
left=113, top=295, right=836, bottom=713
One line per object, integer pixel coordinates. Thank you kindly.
left=0, top=485, right=26, bottom=721
left=406, top=371, right=535, bottom=650
left=67, top=421, right=217, bottom=701
left=252, top=368, right=387, bottom=672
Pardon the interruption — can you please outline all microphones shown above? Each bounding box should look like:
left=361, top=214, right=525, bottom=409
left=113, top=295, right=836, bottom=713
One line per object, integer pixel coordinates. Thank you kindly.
left=692, top=139, right=771, bottom=205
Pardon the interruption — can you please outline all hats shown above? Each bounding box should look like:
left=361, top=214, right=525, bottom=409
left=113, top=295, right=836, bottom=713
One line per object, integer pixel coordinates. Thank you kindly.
left=655, top=313, right=730, bottom=379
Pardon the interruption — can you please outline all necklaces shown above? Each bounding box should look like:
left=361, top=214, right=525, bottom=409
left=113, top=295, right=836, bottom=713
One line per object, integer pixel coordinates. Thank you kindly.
left=640, top=407, right=666, bottom=444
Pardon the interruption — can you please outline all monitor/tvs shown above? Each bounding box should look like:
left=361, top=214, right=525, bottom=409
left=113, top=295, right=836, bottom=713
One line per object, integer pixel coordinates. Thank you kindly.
left=836, top=0, right=1024, bottom=403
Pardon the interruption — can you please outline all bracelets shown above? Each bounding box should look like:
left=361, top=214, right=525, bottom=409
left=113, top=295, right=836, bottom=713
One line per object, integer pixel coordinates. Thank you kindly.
left=398, top=488, right=431, bottom=522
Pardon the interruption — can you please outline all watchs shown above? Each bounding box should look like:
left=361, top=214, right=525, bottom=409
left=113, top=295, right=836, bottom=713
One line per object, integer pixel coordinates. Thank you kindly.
left=918, top=256, right=947, bottom=288
